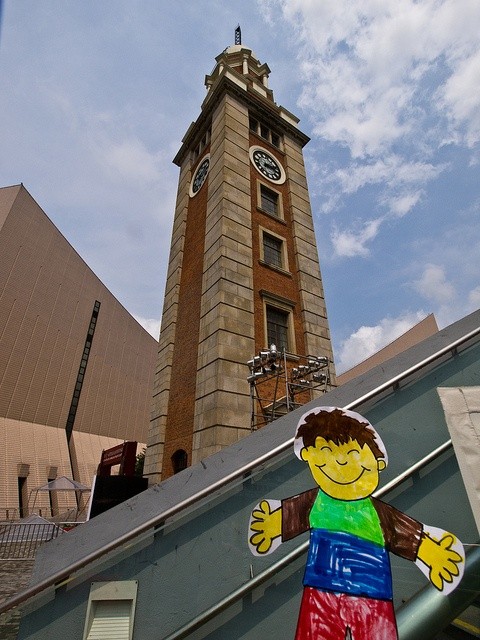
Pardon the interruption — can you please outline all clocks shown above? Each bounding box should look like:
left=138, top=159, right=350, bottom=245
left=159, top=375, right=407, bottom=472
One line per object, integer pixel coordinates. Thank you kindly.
left=250, top=145, right=286, bottom=185
left=188, top=153, right=210, bottom=198
